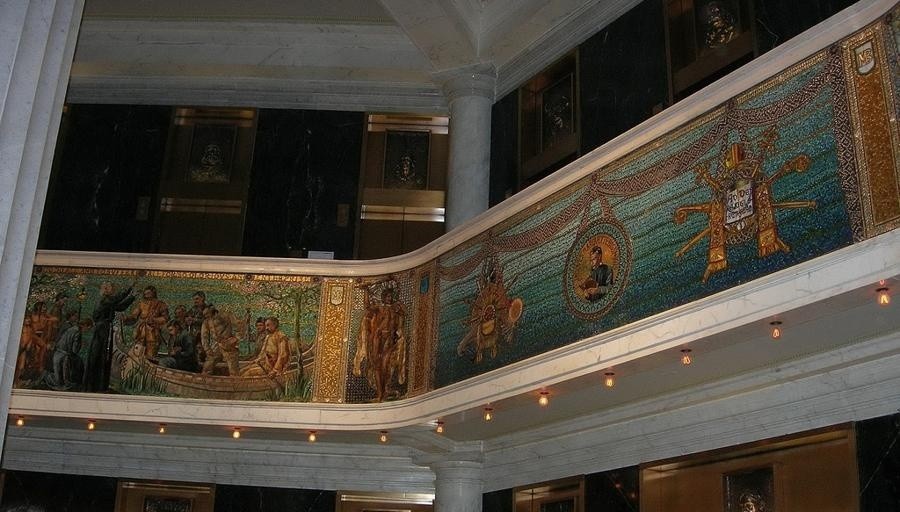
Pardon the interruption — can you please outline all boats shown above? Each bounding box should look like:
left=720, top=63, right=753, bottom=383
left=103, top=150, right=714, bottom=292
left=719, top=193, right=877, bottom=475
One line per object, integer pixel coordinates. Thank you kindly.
left=144, top=357, right=315, bottom=400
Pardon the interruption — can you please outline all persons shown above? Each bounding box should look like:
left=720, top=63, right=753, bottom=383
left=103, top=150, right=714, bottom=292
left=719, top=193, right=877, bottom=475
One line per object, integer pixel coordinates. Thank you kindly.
left=575, top=244, right=615, bottom=307
left=357, top=273, right=408, bottom=403
left=12, top=277, right=291, bottom=394
